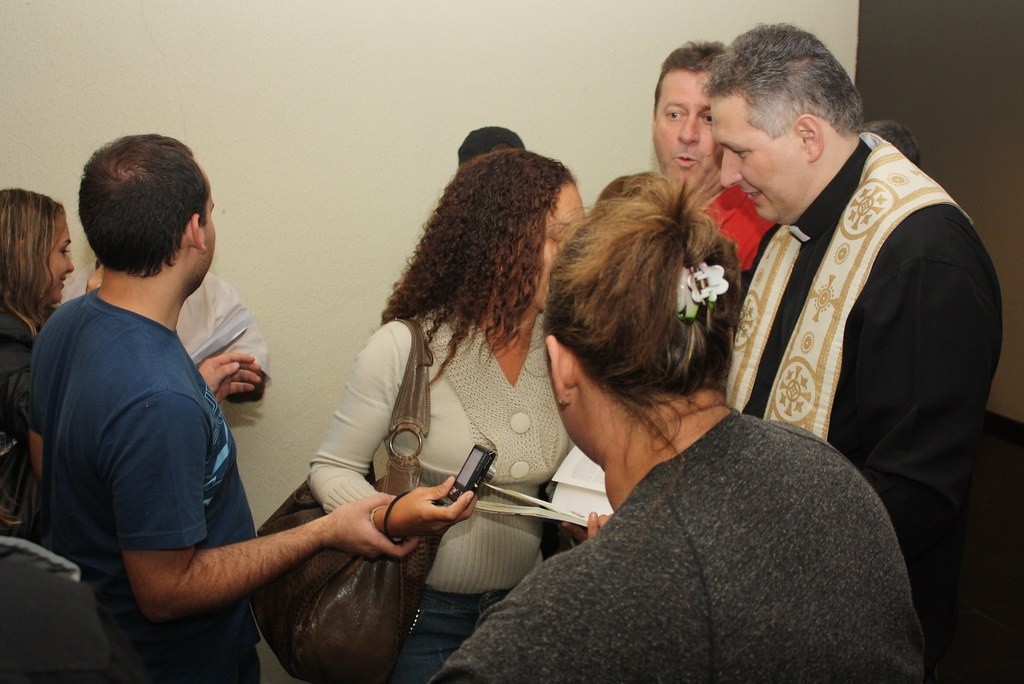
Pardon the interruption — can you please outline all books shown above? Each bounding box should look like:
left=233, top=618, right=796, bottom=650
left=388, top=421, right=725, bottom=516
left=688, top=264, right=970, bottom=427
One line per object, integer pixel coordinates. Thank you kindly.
left=475, top=446, right=613, bottom=527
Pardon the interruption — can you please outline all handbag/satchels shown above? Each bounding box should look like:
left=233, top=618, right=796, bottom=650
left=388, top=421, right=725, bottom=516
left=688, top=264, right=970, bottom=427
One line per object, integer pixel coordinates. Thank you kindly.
left=250, top=318, right=431, bottom=682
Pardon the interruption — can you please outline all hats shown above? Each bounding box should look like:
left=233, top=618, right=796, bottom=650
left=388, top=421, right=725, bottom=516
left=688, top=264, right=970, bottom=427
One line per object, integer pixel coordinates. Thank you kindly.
left=457, top=127, right=525, bottom=166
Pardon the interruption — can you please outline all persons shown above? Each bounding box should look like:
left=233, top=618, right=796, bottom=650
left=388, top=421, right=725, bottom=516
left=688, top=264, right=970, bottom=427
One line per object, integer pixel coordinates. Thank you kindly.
left=308, top=149, right=588, bottom=684
left=61, top=261, right=273, bottom=405
left=0, top=187, right=74, bottom=541
left=653, top=39, right=776, bottom=272
left=28, top=134, right=420, bottom=684
left=423, top=171, right=924, bottom=684
left=458, top=126, right=525, bottom=163
left=559, top=23, right=1004, bottom=669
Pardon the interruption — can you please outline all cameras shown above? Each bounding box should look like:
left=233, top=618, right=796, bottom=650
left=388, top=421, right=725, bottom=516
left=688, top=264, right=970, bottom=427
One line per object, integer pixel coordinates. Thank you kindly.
left=446, top=444, right=496, bottom=508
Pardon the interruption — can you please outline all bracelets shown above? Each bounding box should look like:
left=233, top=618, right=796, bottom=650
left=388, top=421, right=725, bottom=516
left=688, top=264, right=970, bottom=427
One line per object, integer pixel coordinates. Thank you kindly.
left=383, top=491, right=410, bottom=544
